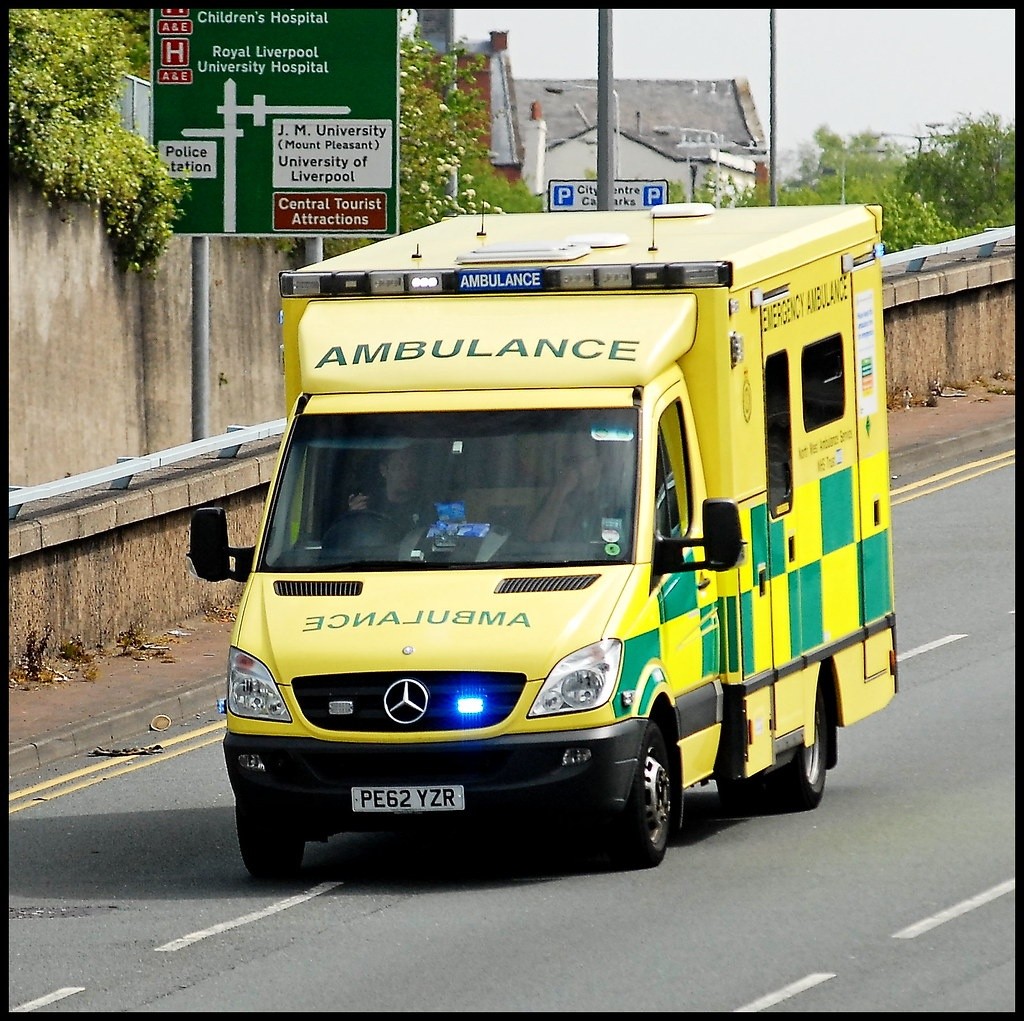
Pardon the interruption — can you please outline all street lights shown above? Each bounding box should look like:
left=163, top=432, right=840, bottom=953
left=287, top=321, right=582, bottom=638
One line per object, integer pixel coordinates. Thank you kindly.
left=839, top=148, right=886, bottom=205
left=873, top=132, right=922, bottom=160
left=544, top=83, right=621, bottom=180
left=653, top=125, right=722, bottom=208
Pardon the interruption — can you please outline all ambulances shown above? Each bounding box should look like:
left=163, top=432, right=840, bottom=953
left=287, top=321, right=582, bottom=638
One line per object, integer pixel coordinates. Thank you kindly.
left=182, top=196, right=901, bottom=879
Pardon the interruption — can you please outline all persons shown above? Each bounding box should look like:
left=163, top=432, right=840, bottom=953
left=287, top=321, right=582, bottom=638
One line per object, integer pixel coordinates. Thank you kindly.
left=527, top=433, right=630, bottom=545
left=349, top=444, right=454, bottom=564
